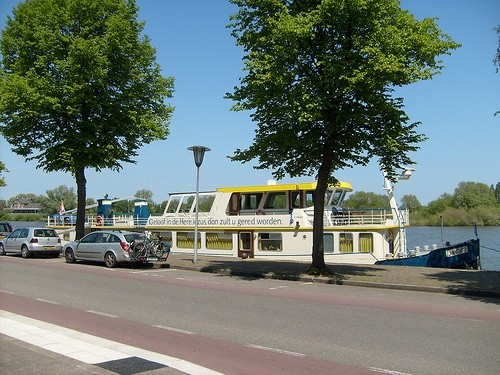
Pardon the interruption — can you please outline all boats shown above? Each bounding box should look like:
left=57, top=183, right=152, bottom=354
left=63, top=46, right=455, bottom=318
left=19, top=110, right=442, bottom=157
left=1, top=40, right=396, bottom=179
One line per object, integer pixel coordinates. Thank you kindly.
left=142, top=179, right=480, bottom=270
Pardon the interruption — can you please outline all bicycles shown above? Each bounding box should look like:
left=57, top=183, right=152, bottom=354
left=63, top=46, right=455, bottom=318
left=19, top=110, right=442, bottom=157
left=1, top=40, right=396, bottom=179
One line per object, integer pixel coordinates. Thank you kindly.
left=128, top=232, right=170, bottom=261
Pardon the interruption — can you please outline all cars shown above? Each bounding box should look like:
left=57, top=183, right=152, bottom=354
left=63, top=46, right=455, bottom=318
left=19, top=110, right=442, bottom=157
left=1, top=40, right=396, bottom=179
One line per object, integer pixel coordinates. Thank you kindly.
left=64, top=232, right=155, bottom=268
left=3, top=227, right=63, bottom=259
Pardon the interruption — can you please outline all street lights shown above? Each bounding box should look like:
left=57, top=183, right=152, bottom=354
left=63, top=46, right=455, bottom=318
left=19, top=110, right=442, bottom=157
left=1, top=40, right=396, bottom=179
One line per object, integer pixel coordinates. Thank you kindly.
left=187, top=145, right=212, bottom=263
